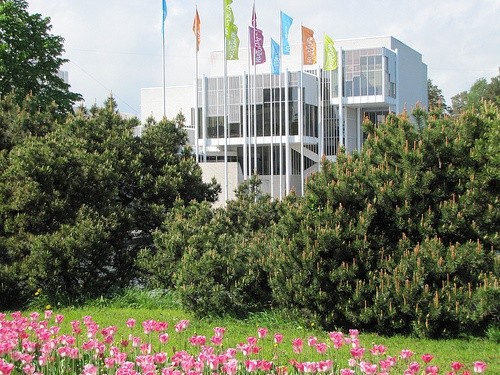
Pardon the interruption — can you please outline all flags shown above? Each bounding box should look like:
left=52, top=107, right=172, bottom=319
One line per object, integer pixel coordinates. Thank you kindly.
left=323, top=32, right=337, bottom=71
left=224, top=0, right=234, bottom=39
left=248, top=27, right=266, bottom=65
left=192, top=11, right=201, bottom=51
left=162, top=0, right=167, bottom=33
left=252, top=5, right=257, bottom=27
left=301, top=26, right=316, bottom=65
left=225, top=24, right=239, bottom=60
left=281, top=12, right=293, bottom=55
left=271, top=39, right=280, bottom=74
